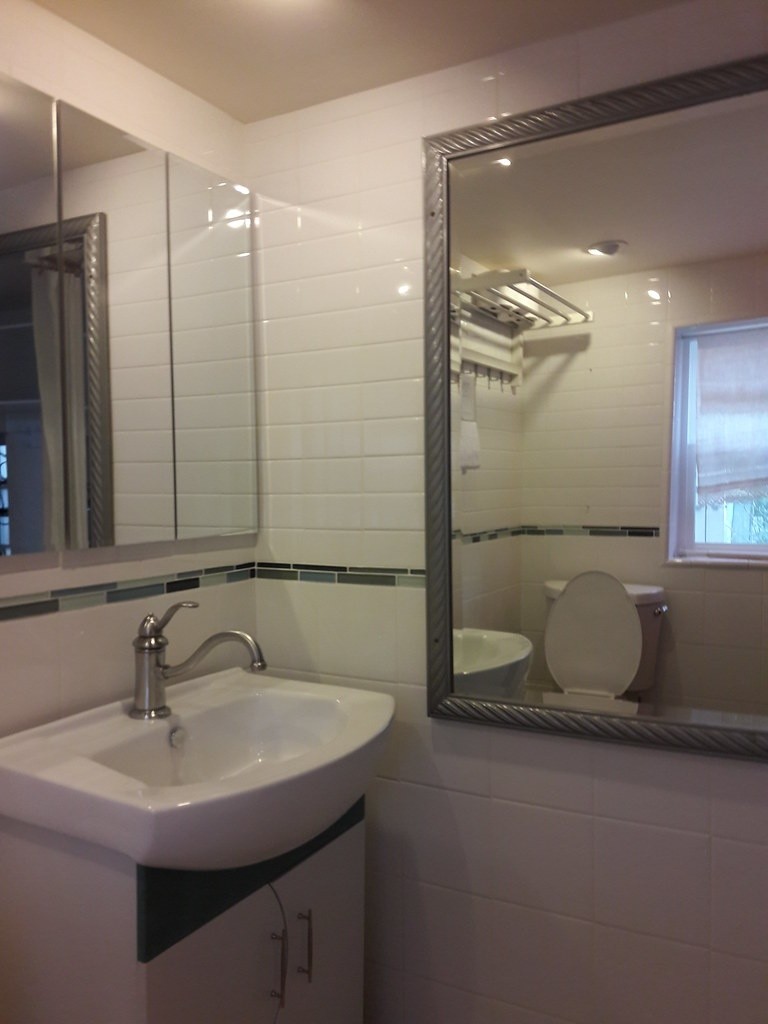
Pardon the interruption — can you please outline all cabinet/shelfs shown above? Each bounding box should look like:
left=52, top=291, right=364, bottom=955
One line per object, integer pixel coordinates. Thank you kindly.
left=2, top=792, right=367, bottom=1022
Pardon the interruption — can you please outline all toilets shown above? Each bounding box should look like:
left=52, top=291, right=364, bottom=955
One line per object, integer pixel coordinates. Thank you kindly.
left=538, top=568, right=665, bottom=722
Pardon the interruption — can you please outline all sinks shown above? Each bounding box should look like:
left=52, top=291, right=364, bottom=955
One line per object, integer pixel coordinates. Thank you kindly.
left=452, top=627, right=534, bottom=703
left=2, top=665, right=396, bottom=872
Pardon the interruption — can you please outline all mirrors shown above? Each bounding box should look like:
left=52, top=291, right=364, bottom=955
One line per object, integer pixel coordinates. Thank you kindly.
left=421, top=51, right=768, bottom=764
left=1, top=71, right=264, bottom=575
left=2, top=212, right=118, bottom=556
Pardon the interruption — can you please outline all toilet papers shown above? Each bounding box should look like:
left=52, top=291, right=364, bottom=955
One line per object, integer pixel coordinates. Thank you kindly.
left=465, top=268, right=540, bottom=330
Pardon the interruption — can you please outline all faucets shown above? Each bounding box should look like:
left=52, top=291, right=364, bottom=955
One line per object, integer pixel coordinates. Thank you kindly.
left=128, top=600, right=268, bottom=722
